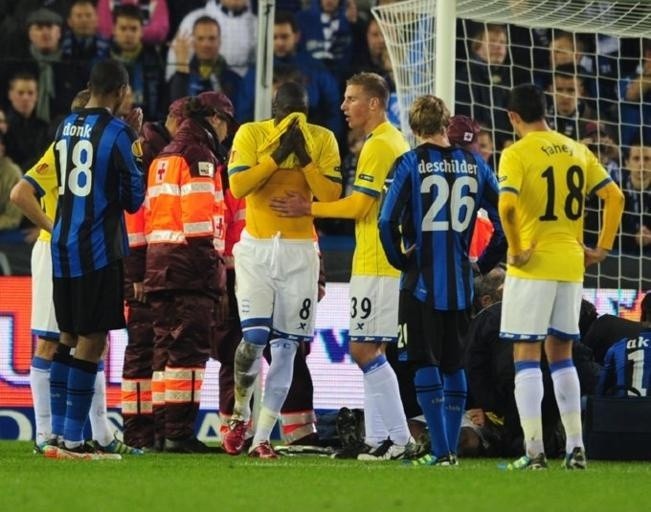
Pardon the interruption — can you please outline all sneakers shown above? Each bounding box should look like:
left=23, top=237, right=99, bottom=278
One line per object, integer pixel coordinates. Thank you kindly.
left=221, top=410, right=252, bottom=455
left=34, top=430, right=144, bottom=461
left=287, top=432, right=337, bottom=455
left=560, top=445, right=590, bottom=470
left=247, top=440, right=281, bottom=461
left=162, top=436, right=219, bottom=455
left=399, top=451, right=460, bottom=469
left=501, top=450, right=552, bottom=472
left=329, top=407, right=419, bottom=461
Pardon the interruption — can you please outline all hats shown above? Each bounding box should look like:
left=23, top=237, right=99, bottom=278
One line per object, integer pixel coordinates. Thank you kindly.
left=24, top=8, right=64, bottom=25
left=197, top=89, right=241, bottom=129
